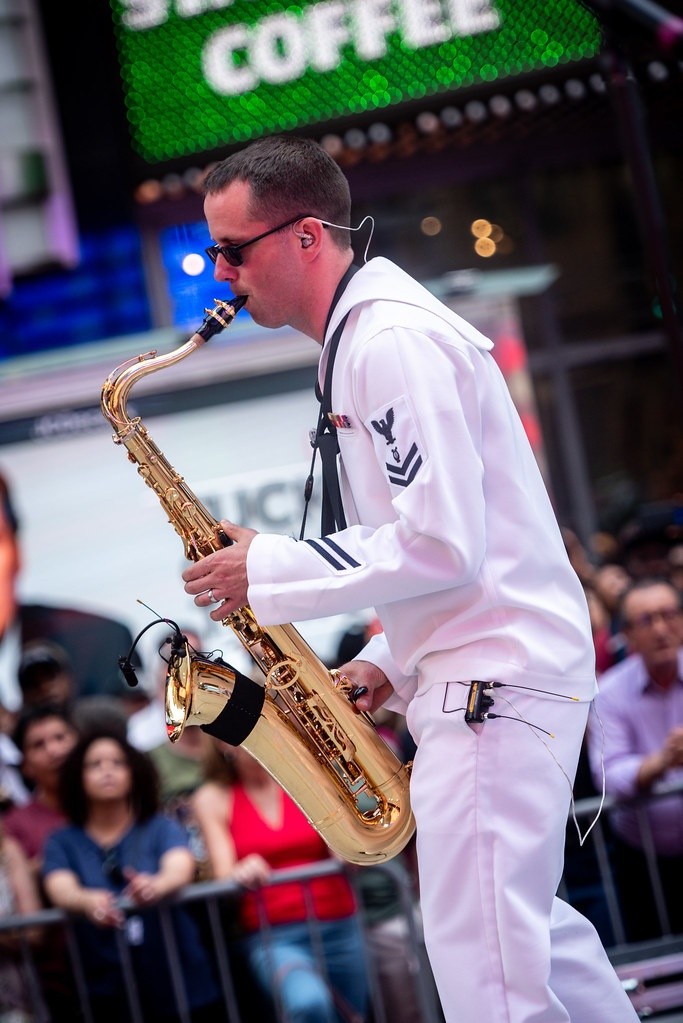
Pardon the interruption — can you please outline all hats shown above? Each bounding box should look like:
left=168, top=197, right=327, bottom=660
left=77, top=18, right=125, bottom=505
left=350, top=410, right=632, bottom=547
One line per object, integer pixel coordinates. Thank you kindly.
left=18, top=640, right=67, bottom=687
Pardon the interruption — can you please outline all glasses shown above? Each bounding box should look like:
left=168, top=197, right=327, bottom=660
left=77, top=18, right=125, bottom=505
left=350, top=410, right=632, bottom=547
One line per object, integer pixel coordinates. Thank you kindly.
left=205, top=212, right=328, bottom=266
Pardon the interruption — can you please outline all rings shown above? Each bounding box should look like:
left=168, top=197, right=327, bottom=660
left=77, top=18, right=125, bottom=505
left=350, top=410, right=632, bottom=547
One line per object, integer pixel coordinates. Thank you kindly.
left=209, top=589, right=219, bottom=604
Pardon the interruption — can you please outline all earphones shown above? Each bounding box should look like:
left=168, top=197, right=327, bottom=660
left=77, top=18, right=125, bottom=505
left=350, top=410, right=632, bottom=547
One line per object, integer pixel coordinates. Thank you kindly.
left=298, top=233, right=314, bottom=248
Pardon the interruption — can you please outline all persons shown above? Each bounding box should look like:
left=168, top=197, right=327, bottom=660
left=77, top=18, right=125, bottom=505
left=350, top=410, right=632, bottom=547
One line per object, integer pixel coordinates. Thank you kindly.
left=182, top=135, right=647, bottom=1023
left=1, top=518, right=683, bottom=1023
left=0, top=472, right=144, bottom=809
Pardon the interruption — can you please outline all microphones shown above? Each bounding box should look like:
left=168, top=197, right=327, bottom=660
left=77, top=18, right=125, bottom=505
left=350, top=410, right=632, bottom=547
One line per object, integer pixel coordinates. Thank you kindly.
left=118, top=619, right=181, bottom=687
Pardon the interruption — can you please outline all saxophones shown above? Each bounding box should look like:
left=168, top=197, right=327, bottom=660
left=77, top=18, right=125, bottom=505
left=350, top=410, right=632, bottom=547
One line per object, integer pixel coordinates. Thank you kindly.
left=100, top=295, right=417, bottom=867
